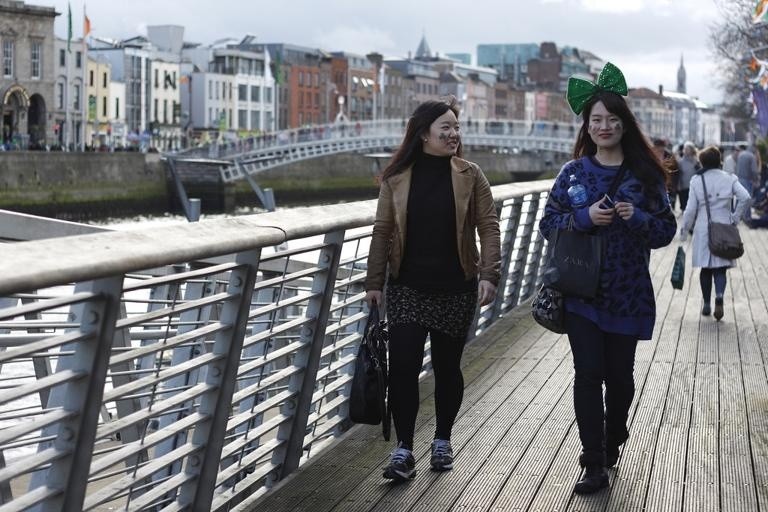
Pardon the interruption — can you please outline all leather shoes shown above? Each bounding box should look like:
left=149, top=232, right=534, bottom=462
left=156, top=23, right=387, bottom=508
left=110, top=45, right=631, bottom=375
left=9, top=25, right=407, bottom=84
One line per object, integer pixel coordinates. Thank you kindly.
left=714, top=296, right=723, bottom=321
left=702, top=307, right=711, bottom=315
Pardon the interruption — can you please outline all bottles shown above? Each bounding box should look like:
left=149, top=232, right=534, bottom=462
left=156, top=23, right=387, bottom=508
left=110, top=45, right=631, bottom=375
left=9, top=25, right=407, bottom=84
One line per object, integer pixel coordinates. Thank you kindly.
left=565, top=173, right=590, bottom=211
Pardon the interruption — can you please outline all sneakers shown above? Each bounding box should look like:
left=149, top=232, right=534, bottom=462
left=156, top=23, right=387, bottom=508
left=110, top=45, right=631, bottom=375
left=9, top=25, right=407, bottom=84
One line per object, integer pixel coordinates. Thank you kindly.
left=382, top=441, right=416, bottom=481
left=430, top=439, right=454, bottom=471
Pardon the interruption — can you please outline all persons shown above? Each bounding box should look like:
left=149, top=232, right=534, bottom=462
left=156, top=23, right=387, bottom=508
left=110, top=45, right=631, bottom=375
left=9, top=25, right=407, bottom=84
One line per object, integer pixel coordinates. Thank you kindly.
left=678, top=147, right=754, bottom=323
left=650, top=138, right=768, bottom=229
left=365, top=100, right=501, bottom=480
left=538, top=61, right=677, bottom=497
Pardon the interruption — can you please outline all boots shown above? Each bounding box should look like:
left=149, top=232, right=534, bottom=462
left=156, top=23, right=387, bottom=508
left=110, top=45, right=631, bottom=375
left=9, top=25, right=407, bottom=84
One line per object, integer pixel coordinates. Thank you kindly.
left=574, top=452, right=609, bottom=493
left=607, top=427, right=629, bottom=468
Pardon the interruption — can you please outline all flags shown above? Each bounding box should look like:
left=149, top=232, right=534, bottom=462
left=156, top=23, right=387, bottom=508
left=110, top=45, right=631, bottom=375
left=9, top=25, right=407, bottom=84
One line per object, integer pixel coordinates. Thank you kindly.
left=84, top=15, right=91, bottom=39
left=67, top=5, right=73, bottom=54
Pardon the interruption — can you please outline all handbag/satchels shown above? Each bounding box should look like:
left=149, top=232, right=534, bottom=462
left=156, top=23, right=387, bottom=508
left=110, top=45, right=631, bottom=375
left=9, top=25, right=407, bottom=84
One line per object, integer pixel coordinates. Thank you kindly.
left=541, top=214, right=604, bottom=300
left=530, top=283, right=568, bottom=334
left=707, top=223, right=743, bottom=259
left=671, top=246, right=685, bottom=290
left=349, top=295, right=391, bottom=442
